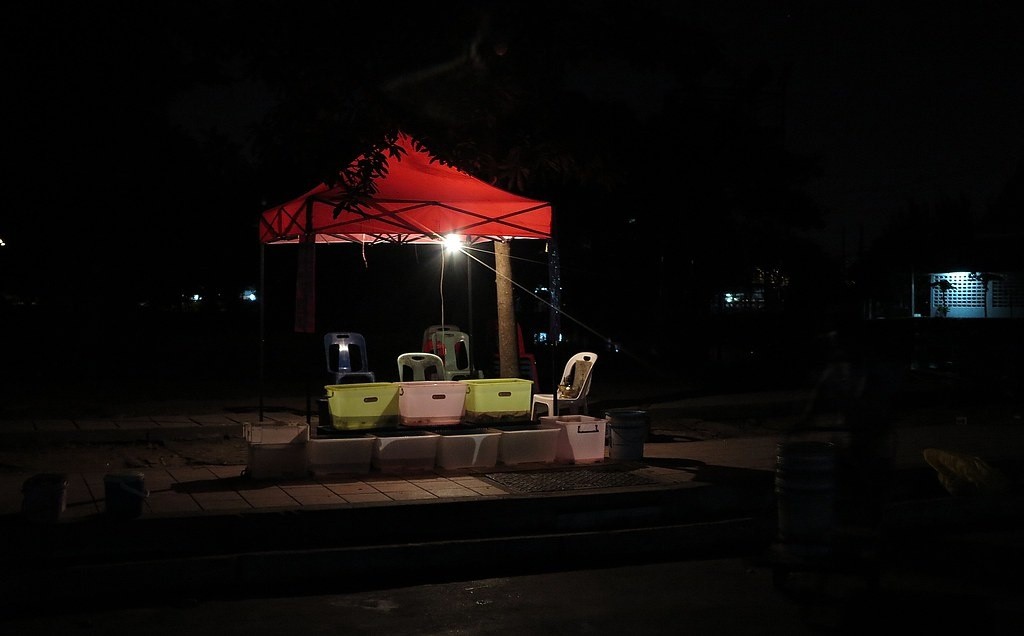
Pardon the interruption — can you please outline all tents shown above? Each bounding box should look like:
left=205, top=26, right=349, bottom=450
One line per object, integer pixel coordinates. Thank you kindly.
left=258, top=128, right=549, bottom=424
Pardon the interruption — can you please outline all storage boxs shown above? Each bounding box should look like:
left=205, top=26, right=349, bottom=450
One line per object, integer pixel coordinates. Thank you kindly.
left=240, top=418, right=608, bottom=479
left=323, top=378, right=534, bottom=431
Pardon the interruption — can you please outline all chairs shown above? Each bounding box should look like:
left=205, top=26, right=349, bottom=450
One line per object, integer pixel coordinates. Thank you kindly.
left=397, top=325, right=484, bottom=382
left=492, top=321, right=540, bottom=393
left=531, top=351, right=599, bottom=419
left=323, top=331, right=376, bottom=384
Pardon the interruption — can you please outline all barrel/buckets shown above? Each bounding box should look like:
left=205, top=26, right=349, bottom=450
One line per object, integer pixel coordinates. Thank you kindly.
left=776, top=441, right=838, bottom=568
left=102, top=470, right=145, bottom=518
left=21, top=474, right=67, bottom=524
left=606, top=410, right=648, bottom=461
